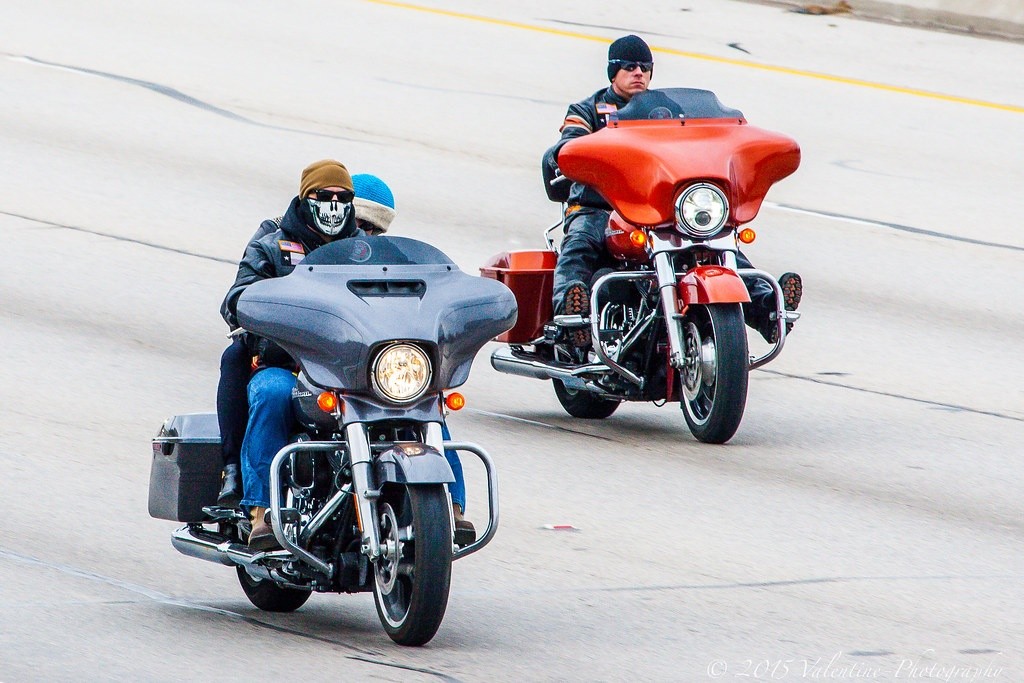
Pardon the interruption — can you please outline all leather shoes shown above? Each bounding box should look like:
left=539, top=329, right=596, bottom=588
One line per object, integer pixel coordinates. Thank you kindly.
left=217, top=463, right=243, bottom=510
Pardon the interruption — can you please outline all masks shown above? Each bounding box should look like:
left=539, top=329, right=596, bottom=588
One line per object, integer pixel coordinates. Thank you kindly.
left=306, top=198, right=354, bottom=237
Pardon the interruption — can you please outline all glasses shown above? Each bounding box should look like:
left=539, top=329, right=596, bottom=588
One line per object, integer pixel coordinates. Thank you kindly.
left=356, top=221, right=384, bottom=237
left=609, top=59, right=654, bottom=73
left=310, top=189, right=354, bottom=205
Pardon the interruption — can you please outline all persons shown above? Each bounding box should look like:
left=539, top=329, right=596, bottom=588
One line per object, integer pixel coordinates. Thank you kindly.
left=226, top=158, right=418, bottom=474
left=553, top=35, right=803, bottom=347
left=218, top=173, right=477, bottom=552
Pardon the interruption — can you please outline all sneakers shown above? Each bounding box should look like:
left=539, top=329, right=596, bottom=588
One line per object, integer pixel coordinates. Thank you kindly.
left=246, top=507, right=272, bottom=544
left=451, top=503, right=475, bottom=545
left=560, top=279, right=593, bottom=350
left=757, top=272, right=803, bottom=345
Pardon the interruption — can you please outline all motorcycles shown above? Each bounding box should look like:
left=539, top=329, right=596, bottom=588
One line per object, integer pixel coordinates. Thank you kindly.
left=478, top=87, right=801, bottom=446
left=148, top=237, right=520, bottom=645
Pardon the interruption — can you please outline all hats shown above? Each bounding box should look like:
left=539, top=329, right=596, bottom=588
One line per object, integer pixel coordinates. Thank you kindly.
left=348, top=172, right=396, bottom=232
left=606, top=35, right=654, bottom=82
left=298, top=159, right=355, bottom=198
